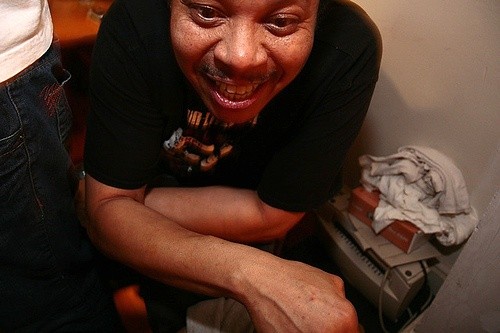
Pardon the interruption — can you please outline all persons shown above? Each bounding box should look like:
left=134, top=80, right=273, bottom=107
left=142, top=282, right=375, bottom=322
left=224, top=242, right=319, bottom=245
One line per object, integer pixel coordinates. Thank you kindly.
left=76, top=0, right=382, bottom=333
left=0, top=0, right=112, bottom=333
